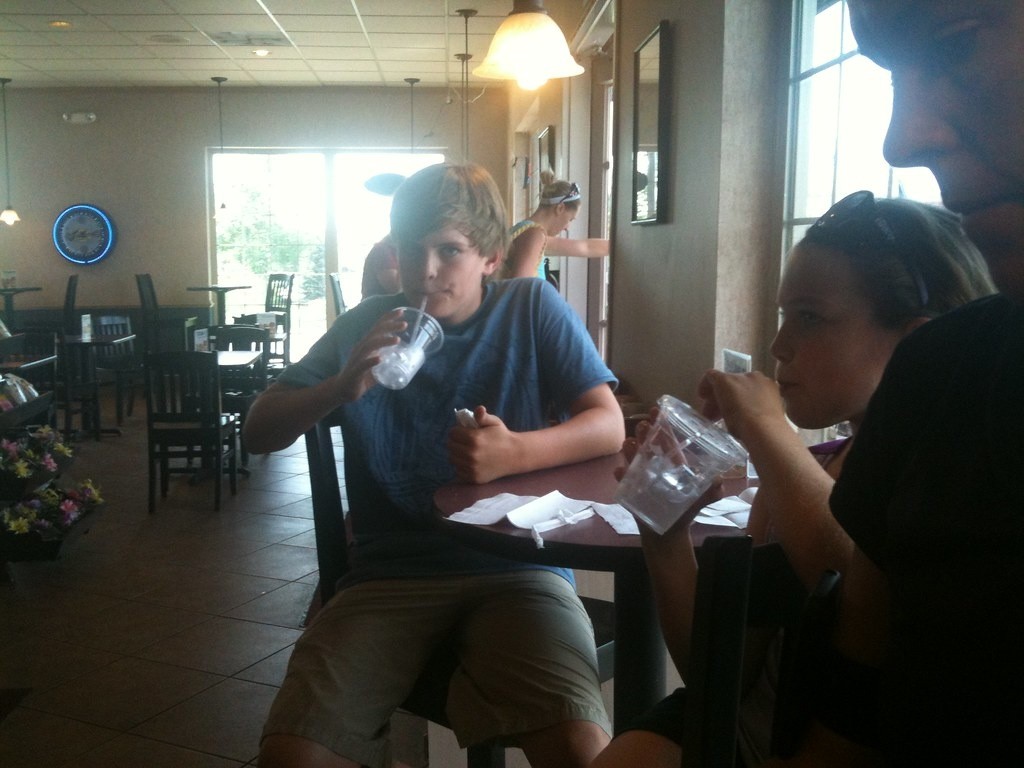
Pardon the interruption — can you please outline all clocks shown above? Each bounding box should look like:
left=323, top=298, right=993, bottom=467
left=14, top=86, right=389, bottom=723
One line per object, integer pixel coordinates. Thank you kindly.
left=52, top=204, right=115, bottom=265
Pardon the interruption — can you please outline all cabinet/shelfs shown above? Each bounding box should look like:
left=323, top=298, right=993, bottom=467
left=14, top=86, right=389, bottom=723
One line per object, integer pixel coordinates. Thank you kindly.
left=0, top=391, right=99, bottom=586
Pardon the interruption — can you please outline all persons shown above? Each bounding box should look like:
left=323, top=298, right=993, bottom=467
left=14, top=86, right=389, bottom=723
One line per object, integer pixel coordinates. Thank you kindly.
left=489, top=170, right=611, bottom=279
left=589, top=190, right=998, bottom=768
left=243, top=161, right=627, bottom=767
left=759, top=0, right=1024, bottom=768
left=362, top=231, right=403, bottom=298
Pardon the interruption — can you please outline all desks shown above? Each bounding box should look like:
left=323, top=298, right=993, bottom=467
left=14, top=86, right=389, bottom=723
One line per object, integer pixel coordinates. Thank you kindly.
left=57, top=333, right=138, bottom=441
left=186, top=284, right=252, bottom=325
left=164, top=348, right=263, bottom=479
left=0, top=354, right=56, bottom=385
left=435, top=435, right=761, bottom=736
left=0, top=287, right=43, bottom=328
left=208, top=331, right=289, bottom=352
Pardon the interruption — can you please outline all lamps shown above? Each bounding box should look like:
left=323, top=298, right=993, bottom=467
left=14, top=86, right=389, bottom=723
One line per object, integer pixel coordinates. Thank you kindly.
left=62, top=113, right=96, bottom=125
left=210, top=77, right=230, bottom=220
left=0, top=78, right=22, bottom=225
left=472, top=0, right=585, bottom=90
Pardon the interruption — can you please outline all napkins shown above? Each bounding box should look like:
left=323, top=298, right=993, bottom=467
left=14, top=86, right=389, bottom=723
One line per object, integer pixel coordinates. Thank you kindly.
left=442, top=489, right=752, bottom=549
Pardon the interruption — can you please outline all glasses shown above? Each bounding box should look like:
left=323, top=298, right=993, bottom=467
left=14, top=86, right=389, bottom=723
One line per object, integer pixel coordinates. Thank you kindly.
left=560, top=183, right=580, bottom=202
left=805, top=191, right=928, bottom=311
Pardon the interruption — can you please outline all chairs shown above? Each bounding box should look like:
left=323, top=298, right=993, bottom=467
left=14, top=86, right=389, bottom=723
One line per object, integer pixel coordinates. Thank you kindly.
left=680, top=532, right=842, bottom=768
left=302, top=408, right=614, bottom=768
left=0, top=274, right=296, bottom=516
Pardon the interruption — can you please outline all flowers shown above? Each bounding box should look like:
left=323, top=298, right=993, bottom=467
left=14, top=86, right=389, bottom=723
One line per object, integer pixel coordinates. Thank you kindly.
left=1, top=476, right=107, bottom=543
left=0, top=422, right=78, bottom=480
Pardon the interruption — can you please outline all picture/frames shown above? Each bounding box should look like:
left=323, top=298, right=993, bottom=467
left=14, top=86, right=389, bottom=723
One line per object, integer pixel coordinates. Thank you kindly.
left=538, top=125, right=555, bottom=201
left=630, top=19, right=667, bottom=224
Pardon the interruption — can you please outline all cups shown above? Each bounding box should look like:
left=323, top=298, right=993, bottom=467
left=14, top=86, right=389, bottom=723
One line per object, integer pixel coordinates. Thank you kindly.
left=369, top=306, right=443, bottom=390
left=614, top=394, right=748, bottom=536
